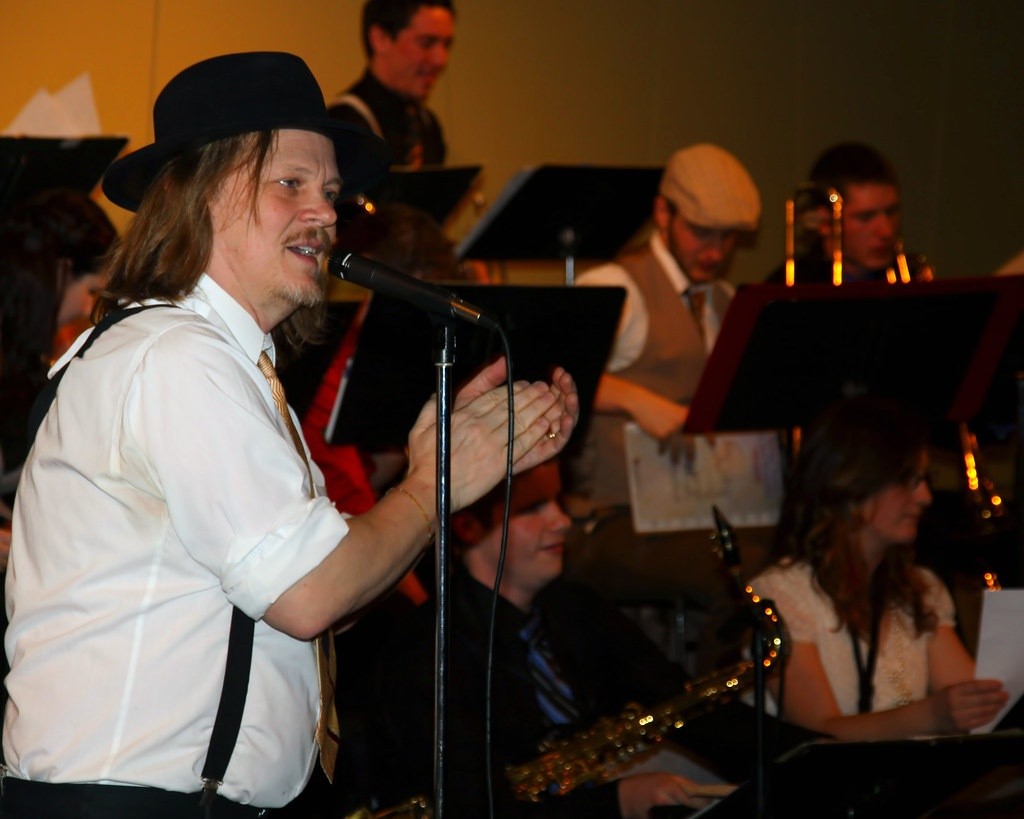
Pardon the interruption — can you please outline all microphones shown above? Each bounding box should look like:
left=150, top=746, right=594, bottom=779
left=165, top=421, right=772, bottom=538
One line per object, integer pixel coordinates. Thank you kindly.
left=712, top=509, right=751, bottom=609
left=328, top=249, right=500, bottom=330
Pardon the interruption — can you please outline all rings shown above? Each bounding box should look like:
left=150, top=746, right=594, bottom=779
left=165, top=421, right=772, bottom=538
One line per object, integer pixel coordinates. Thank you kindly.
left=545, top=430, right=555, bottom=439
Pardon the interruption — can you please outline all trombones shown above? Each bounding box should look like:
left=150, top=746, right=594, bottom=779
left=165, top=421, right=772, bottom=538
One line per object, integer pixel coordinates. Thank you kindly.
left=782, top=179, right=844, bottom=469
left=883, top=240, right=1003, bottom=590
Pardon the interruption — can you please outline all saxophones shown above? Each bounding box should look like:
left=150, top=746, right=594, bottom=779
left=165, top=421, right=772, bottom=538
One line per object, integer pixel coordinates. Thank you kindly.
left=343, top=504, right=793, bottom=819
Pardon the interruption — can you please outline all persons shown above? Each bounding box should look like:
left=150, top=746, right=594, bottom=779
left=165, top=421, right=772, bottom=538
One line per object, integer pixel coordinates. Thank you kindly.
left=0, top=51, right=578, bottom=819
left=740, top=398, right=1008, bottom=741
left=0, top=192, right=119, bottom=395
left=766, top=142, right=1024, bottom=284
left=591, top=142, right=762, bottom=438
left=330, top=202, right=465, bottom=284
left=322, top=0, right=456, bottom=171
left=373, top=458, right=709, bottom=819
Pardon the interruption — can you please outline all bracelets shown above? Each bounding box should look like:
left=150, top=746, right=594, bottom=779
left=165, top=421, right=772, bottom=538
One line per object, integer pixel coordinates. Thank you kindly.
left=386, top=488, right=432, bottom=539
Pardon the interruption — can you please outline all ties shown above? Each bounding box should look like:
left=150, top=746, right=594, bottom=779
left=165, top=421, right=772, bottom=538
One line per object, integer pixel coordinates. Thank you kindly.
left=688, top=289, right=707, bottom=354
left=408, top=105, right=423, bottom=168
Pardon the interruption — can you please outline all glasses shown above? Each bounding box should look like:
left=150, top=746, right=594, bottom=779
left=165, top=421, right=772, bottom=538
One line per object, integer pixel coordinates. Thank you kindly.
left=899, top=467, right=934, bottom=490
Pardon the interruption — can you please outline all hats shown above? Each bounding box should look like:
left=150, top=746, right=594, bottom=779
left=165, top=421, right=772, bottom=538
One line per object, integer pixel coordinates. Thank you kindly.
left=100, top=51, right=387, bottom=214
left=660, top=141, right=761, bottom=233
